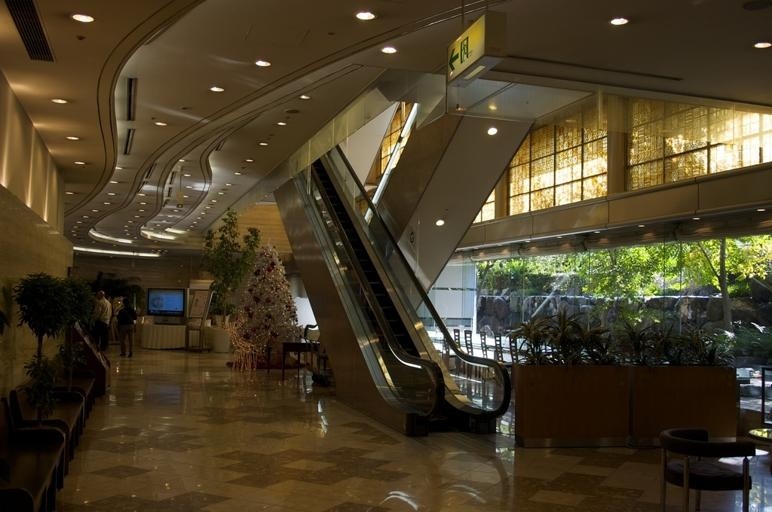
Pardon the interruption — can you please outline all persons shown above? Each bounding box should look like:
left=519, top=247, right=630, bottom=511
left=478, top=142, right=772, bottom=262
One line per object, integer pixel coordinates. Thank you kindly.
left=117, top=298, right=138, bottom=359
left=93, top=290, right=113, bottom=352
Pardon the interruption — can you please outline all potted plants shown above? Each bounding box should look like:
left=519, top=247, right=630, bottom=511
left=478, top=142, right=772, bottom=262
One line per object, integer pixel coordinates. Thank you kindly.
left=197, top=204, right=263, bottom=352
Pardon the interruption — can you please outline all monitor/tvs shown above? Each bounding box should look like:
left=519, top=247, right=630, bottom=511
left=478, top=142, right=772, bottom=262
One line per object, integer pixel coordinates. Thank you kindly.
left=146, top=287, right=185, bottom=317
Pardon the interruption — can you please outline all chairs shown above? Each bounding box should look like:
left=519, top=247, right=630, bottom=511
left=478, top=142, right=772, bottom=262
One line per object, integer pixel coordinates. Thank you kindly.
left=658, top=428, right=753, bottom=512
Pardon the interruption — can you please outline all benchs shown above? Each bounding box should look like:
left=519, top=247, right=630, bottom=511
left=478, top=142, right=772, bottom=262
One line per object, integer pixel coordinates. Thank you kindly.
left=0, top=368, right=98, bottom=512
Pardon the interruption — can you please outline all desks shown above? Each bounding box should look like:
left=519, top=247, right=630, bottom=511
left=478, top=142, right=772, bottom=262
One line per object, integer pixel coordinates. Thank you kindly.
left=141, top=324, right=186, bottom=350
left=744, top=428, right=772, bottom=452
left=281, top=342, right=320, bottom=384
